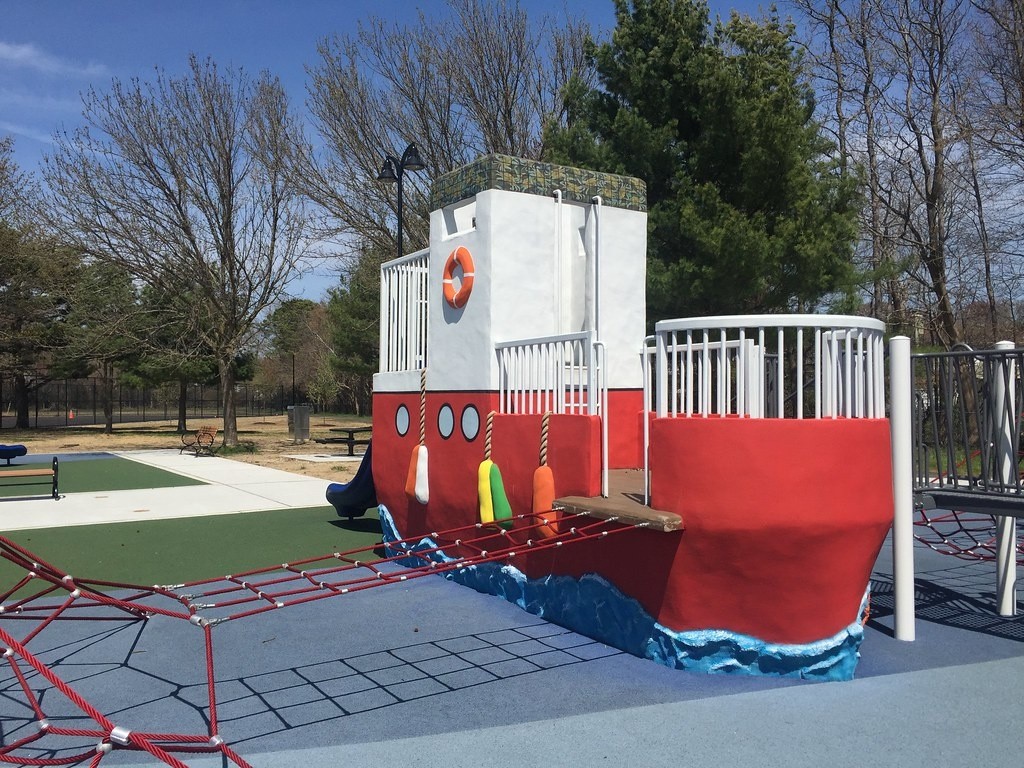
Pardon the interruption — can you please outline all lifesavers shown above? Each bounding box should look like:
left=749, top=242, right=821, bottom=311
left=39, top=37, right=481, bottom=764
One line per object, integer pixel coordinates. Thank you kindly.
left=441, top=246, right=475, bottom=312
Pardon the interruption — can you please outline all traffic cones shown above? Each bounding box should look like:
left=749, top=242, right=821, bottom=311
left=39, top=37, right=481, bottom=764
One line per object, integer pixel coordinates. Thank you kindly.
left=68, top=409, right=75, bottom=420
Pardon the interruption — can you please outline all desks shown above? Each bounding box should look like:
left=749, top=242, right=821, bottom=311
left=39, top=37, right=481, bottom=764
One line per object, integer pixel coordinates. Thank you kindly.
left=329, top=427, right=372, bottom=456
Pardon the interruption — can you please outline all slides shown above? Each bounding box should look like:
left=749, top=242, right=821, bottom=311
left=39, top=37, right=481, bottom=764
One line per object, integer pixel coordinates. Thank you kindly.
left=326, top=440, right=379, bottom=522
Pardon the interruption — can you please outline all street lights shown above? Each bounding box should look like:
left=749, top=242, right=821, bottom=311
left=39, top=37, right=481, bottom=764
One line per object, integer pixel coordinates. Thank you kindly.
left=376, top=140, right=427, bottom=258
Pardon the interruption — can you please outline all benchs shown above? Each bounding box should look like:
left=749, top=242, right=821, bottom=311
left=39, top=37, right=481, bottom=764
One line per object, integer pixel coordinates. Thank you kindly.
left=313, top=438, right=370, bottom=444
left=0, top=457, right=59, bottom=501
left=179, top=426, right=218, bottom=458
